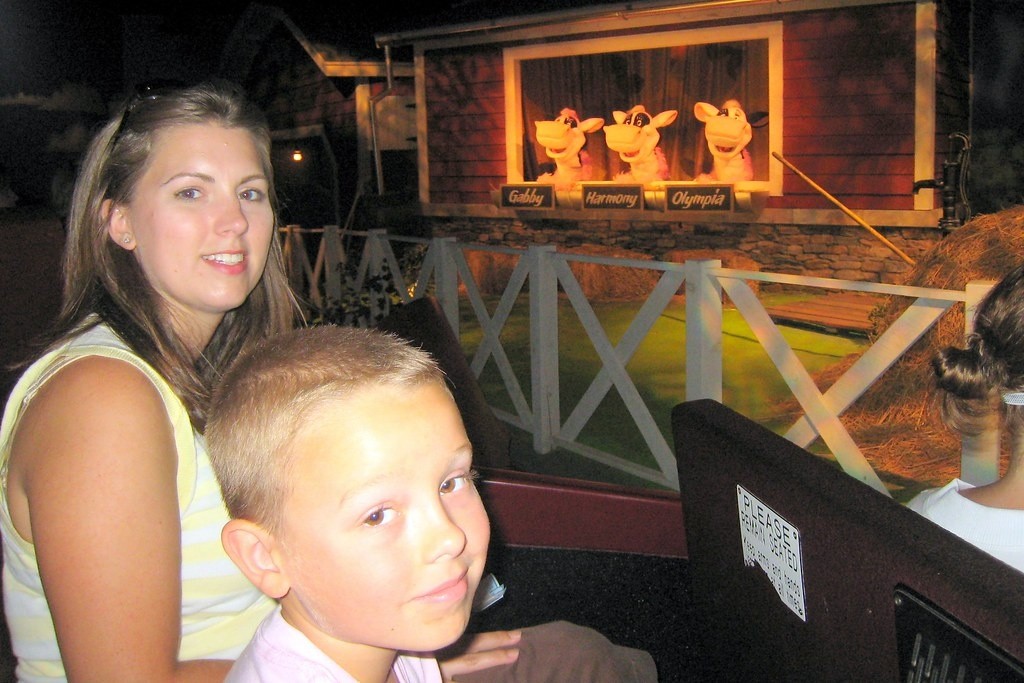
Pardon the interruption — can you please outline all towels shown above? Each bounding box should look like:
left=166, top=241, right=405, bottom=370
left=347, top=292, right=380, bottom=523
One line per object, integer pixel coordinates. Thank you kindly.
left=374, top=295, right=512, bottom=472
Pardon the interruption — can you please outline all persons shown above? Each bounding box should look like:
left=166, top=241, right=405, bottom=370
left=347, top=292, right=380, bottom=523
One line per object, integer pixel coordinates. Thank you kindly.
left=0, top=82, right=659, bottom=683
left=204, top=325, right=491, bottom=683
left=905, top=264, right=1024, bottom=572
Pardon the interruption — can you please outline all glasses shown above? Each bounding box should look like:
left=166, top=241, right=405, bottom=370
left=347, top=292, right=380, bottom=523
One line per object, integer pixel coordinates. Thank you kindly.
left=111, top=73, right=212, bottom=154
